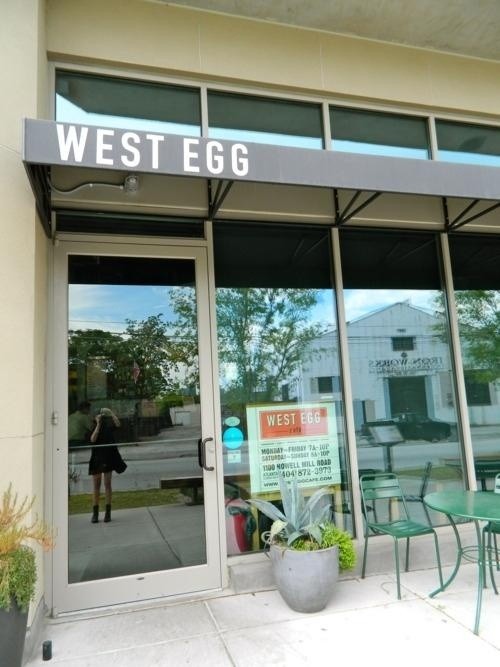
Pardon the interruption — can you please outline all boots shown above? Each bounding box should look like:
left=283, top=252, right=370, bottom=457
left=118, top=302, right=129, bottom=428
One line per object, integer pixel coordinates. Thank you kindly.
left=92, top=505, right=98, bottom=522
left=104, top=504, right=111, bottom=522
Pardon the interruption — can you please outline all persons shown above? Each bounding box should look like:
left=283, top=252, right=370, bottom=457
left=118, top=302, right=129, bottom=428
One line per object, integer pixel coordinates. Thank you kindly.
left=86, top=407, right=126, bottom=522
left=69, top=401, right=93, bottom=443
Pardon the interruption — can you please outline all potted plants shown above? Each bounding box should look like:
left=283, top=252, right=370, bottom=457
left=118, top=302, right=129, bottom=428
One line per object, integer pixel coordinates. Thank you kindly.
left=244, top=462, right=358, bottom=613
left=0, top=481, right=60, bottom=667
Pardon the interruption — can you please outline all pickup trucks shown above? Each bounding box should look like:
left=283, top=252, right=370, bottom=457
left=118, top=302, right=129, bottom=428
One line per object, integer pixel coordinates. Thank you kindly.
left=361, top=409, right=454, bottom=444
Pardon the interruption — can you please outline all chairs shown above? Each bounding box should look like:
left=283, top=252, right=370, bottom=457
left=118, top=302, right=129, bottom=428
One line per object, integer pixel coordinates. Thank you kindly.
left=482, top=522, right=498, bottom=593
left=389, top=461, right=432, bottom=526
left=360, top=470, right=445, bottom=597
left=329, top=469, right=379, bottom=537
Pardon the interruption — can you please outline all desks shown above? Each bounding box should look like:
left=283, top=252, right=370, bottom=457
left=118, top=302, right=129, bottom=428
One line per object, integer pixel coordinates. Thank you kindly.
left=422, top=490, right=499, bottom=637
left=228, top=478, right=344, bottom=551
